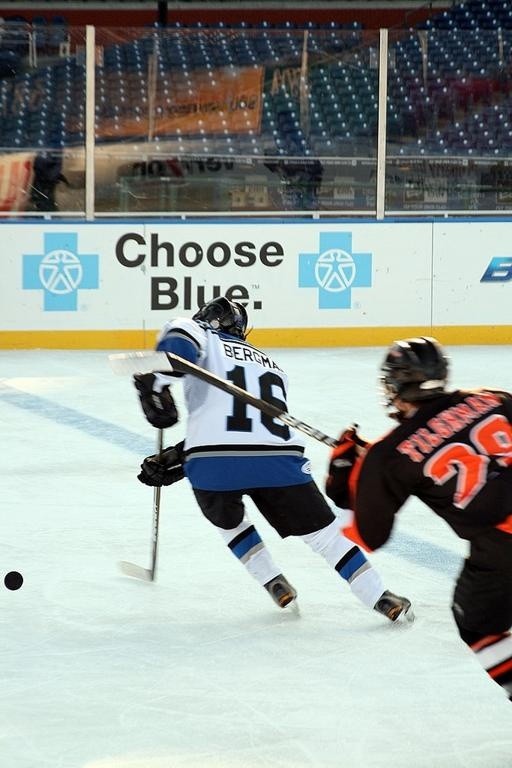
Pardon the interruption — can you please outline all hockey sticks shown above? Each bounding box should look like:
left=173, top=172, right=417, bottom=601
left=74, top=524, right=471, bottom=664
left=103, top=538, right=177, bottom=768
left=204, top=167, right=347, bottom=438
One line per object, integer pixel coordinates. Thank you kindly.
left=119, top=429, right=163, bottom=582
left=108, top=350, right=339, bottom=450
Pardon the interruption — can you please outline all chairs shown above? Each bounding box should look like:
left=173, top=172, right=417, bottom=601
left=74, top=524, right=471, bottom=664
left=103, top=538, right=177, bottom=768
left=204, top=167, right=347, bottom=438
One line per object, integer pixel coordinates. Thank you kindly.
left=7, top=1, right=511, bottom=178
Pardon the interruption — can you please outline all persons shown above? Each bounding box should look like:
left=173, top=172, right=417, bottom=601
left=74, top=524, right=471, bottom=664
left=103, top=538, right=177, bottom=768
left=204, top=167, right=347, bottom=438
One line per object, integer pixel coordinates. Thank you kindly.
left=133, top=295, right=413, bottom=623
left=325, top=336, right=511, bottom=703
left=30, top=126, right=71, bottom=211
left=265, top=113, right=323, bottom=211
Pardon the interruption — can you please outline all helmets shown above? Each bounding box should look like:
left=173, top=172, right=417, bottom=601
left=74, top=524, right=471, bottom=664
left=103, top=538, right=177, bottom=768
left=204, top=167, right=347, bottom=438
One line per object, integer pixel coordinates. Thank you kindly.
left=377, top=335, right=449, bottom=424
left=192, top=296, right=249, bottom=340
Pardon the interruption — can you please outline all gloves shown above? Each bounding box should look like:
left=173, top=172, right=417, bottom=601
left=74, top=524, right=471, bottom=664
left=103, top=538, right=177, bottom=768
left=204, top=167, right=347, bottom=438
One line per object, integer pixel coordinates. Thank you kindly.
left=325, top=428, right=364, bottom=511
left=133, top=372, right=178, bottom=427
left=137, top=439, right=185, bottom=486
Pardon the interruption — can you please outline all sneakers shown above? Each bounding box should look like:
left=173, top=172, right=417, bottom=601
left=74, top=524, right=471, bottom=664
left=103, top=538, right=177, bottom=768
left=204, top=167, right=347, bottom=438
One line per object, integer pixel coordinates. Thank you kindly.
left=263, top=573, right=297, bottom=608
left=373, top=589, right=410, bottom=621
